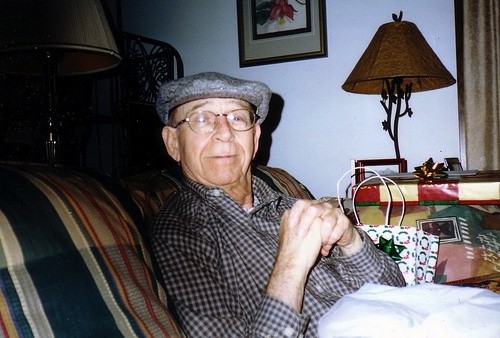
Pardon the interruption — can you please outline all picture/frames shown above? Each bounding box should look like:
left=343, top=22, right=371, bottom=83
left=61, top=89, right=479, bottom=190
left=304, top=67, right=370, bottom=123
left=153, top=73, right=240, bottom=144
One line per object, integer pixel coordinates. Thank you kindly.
left=236, top=0, right=329, bottom=69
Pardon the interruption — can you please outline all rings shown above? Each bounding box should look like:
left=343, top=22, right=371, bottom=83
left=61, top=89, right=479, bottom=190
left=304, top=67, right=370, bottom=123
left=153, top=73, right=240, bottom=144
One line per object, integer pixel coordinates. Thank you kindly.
left=318, top=215, right=325, bottom=221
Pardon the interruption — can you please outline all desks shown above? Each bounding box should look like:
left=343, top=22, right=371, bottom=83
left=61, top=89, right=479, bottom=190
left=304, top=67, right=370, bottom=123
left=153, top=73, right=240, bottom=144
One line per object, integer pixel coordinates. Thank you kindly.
left=351, top=170, right=500, bottom=206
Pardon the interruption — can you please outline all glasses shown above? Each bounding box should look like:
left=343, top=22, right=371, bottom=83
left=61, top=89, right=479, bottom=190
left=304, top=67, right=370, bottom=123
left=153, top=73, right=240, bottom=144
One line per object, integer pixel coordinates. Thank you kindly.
left=173, top=109, right=259, bottom=133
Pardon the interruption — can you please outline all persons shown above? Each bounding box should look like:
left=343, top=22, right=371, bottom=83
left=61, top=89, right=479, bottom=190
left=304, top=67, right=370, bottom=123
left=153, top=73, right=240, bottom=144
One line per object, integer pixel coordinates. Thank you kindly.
left=140, top=72, right=406, bottom=338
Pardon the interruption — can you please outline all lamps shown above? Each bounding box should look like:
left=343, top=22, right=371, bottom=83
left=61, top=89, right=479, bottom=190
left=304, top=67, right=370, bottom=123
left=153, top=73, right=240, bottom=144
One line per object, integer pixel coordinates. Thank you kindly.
left=0, top=0, right=123, bottom=157
left=340, top=10, right=458, bottom=159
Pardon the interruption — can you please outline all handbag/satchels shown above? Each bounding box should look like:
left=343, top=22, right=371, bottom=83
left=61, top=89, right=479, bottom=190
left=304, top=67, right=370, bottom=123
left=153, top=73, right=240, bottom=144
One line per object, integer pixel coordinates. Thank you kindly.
left=335, top=164, right=439, bottom=288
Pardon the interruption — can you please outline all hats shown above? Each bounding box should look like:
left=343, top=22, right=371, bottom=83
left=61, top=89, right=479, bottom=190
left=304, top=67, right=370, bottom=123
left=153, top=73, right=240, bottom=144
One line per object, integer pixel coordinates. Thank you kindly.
left=156, top=70, right=271, bottom=127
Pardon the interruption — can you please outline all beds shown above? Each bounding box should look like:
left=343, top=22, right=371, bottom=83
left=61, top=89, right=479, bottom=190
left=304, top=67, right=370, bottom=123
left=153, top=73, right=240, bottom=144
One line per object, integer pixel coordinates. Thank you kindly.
left=0, top=164, right=500, bottom=338
